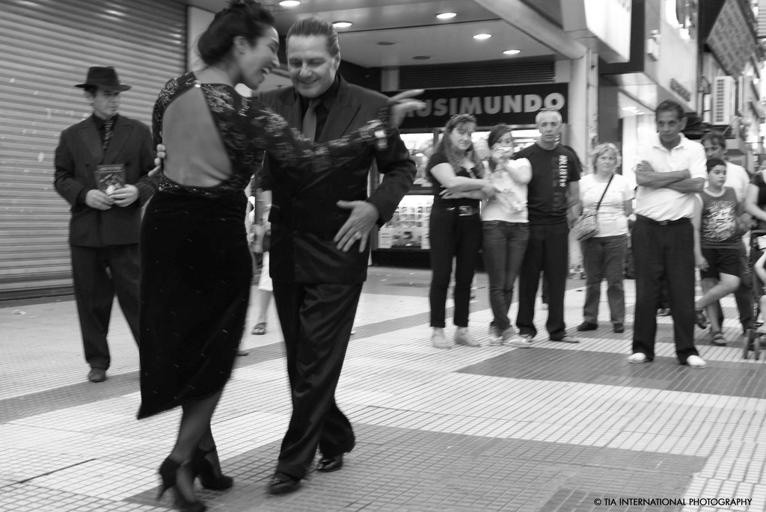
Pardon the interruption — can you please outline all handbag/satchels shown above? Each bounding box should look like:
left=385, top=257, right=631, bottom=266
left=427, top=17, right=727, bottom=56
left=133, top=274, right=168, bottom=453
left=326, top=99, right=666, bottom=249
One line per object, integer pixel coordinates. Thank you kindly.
left=572, top=174, right=615, bottom=243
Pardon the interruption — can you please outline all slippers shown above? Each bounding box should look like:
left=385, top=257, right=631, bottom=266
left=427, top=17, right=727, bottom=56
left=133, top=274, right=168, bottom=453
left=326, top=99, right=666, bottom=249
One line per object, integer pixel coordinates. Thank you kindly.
left=251, top=322, right=266, bottom=335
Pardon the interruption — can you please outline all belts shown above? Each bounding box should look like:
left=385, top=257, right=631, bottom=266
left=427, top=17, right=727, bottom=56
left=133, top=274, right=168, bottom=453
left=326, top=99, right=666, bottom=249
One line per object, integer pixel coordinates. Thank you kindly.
left=635, top=214, right=690, bottom=227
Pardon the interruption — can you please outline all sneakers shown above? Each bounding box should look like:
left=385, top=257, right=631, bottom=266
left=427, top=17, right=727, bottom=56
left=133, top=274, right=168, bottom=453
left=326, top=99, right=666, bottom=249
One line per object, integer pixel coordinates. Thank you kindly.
left=430, top=321, right=627, bottom=349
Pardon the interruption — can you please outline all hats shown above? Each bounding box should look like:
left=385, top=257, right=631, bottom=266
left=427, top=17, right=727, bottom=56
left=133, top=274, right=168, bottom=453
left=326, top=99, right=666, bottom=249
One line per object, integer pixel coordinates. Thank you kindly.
left=74, top=64, right=131, bottom=93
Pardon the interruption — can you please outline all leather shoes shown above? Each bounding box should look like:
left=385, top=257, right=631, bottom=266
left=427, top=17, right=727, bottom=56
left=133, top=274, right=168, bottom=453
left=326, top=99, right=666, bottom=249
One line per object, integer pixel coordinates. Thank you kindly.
left=627, top=351, right=652, bottom=363
left=87, top=367, right=107, bottom=382
left=685, top=354, right=706, bottom=369
left=315, top=423, right=356, bottom=473
left=268, top=467, right=303, bottom=493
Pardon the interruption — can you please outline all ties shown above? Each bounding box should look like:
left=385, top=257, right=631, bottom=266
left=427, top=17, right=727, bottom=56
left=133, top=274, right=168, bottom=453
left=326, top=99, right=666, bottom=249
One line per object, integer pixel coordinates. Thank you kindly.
left=103, top=118, right=114, bottom=150
left=302, top=99, right=318, bottom=146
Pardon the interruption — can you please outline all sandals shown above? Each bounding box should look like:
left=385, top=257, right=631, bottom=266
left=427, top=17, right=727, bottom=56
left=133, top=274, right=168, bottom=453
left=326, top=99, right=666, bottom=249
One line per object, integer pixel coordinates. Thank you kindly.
left=693, top=308, right=707, bottom=329
left=711, top=331, right=726, bottom=346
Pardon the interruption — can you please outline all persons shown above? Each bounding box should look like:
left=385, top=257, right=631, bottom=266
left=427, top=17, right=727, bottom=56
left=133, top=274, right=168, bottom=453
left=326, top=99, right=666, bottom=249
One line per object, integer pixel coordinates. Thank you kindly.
left=53, top=1, right=765, bottom=512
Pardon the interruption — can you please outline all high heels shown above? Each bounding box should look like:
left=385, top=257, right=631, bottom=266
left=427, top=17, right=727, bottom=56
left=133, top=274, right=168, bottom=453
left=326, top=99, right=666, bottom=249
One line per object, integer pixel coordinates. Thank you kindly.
left=155, top=456, right=206, bottom=511
left=195, top=442, right=235, bottom=491
